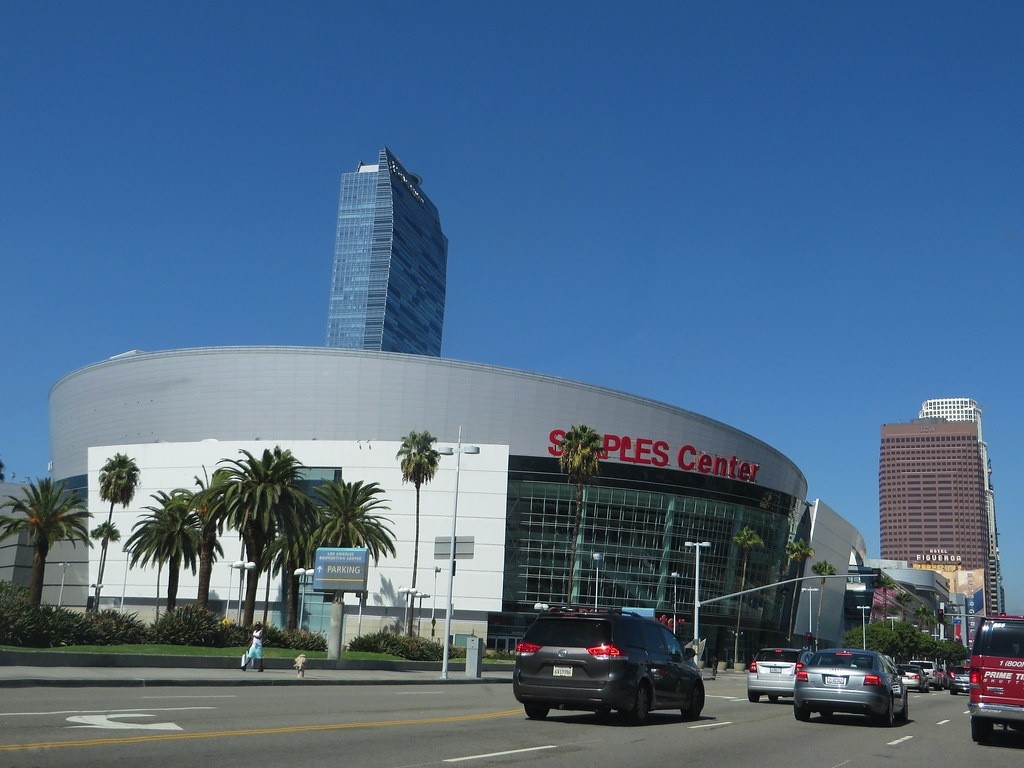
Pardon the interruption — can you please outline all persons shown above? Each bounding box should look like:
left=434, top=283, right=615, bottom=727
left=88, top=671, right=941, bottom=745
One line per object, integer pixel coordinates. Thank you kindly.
left=241, top=624, right=264, bottom=672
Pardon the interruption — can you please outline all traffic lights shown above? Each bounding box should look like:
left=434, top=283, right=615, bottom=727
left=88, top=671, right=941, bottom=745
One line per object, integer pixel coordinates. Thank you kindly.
left=937, top=609, right=943, bottom=623
left=1000, top=612, right=1006, bottom=617
left=805, top=632, right=813, bottom=645
left=669, top=592, right=675, bottom=606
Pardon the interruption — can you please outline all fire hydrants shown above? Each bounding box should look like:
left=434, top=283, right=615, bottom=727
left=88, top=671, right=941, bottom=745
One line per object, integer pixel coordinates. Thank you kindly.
left=293, top=654, right=307, bottom=678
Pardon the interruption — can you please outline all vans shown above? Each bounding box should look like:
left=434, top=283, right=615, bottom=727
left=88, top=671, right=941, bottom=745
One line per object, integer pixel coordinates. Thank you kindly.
left=967, top=616, right=1024, bottom=743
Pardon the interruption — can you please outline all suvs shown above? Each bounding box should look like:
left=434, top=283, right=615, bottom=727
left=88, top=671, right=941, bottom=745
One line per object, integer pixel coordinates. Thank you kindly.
left=512, top=606, right=705, bottom=726
left=949, top=665, right=970, bottom=695
left=908, top=660, right=943, bottom=691
left=747, top=648, right=800, bottom=705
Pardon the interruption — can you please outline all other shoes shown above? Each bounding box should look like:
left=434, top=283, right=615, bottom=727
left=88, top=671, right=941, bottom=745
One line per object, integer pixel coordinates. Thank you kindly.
left=258, top=668, right=263, bottom=672
left=240, top=665, right=246, bottom=671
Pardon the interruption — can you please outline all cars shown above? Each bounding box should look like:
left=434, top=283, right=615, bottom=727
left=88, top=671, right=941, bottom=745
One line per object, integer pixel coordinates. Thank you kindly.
left=938, top=668, right=950, bottom=690
left=794, top=648, right=908, bottom=727
left=895, top=664, right=930, bottom=694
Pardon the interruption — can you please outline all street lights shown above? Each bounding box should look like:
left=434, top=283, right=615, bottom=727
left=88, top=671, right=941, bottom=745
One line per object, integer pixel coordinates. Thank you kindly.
left=670, top=572, right=680, bottom=655
left=414, top=592, right=430, bottom=637
left=399, top=587, right=417, bottom=635
left=801, top=587, right=819, bottom=652
left=685, top=541, right=711, bottom=666
left=592, top=553, right=600, bottom=612
left=232, top=560, right=257, bottom=626
left=887, top=617, right=898, bottom=664
left=119, top=548, right=133, bottom=616
left=58, top=561, right=72, bottom=606
left=294, top=567, right=314, bottom=631
left=438, top=424, right=480, bottom=680
left=931, top=634, right=939, bottom=664
left=857, top=605, right=870, bottom=650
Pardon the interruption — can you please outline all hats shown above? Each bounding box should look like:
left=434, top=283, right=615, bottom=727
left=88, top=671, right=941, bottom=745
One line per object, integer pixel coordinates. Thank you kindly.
left=255, top=621, right=260, bottom=625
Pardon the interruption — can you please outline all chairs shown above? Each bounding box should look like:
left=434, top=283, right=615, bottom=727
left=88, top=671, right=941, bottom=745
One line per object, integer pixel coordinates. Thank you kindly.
left=820, top=656, right=837, bottom=666
left=855, top=658, right=872, bottom=669
